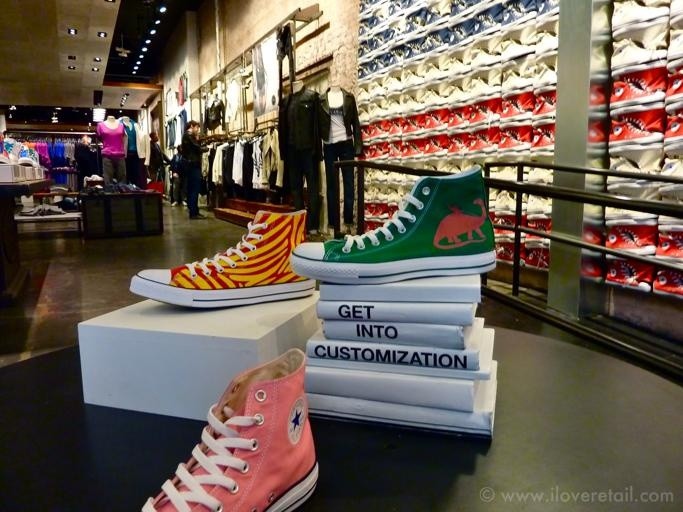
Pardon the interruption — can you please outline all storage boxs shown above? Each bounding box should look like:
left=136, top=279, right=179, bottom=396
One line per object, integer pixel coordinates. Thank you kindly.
left=78, top=289, right=322, bottom=422
left=80, top=189, right=163, bottom=239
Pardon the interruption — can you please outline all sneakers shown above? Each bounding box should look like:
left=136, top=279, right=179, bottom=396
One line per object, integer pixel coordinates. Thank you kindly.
left=128, top=210, right=316, bottom=308
left=0, top=134, right=39, bottom=167
left=191, top=215, right=204, bottom=219
left=292, top=163, right=496, bottom=285
left=139, top=347, right=320, bottom=511
left=582, top=1, right=682, bottom=300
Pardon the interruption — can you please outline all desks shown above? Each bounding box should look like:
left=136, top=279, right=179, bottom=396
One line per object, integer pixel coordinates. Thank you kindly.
left=0, top=322, right=682, bottom=512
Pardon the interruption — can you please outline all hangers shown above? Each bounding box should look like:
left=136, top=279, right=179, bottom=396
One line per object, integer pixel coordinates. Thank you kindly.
left=5, top=132, right=82, bottom=145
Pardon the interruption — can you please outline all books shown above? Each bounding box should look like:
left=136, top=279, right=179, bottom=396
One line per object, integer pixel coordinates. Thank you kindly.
left=295, top=280, right=498, bottom=436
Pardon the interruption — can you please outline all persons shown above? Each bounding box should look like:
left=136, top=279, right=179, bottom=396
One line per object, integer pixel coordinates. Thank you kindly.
left=165, top=144, right=187, bottom=206
left=278, top=81, right=321, bottom=235
left=203, top=94, right=225, bottom=132
left=97, top=116, right=126, bottom=186
left=149, top=131, right=170, bottom=200
left=120, top=116, right=150, bottom=188
left=180, top=121, right=208, bottom=220
left=319, top=79, right=361, bottom=234
left=74, top=136, right=94, bottom=190
